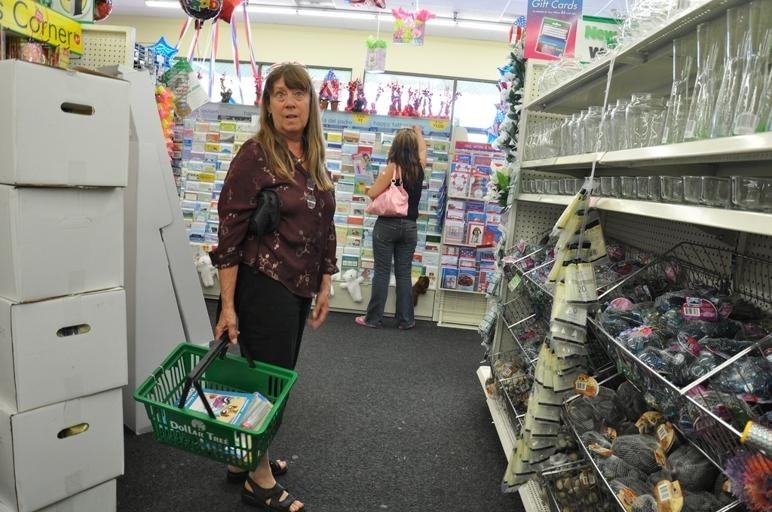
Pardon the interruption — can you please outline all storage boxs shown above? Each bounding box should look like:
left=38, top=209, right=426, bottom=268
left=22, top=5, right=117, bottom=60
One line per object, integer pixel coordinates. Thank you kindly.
left=2, top=288, right=128, bottom=411
left=2, top=388, right=124, bottom=512
left=1, top=183, right=124, bottom=304
left=0, top=58, right=131, bottom=190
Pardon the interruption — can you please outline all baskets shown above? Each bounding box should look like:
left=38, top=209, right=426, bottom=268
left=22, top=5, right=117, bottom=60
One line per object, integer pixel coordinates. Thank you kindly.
left=134, top=329, right=299, bottom=472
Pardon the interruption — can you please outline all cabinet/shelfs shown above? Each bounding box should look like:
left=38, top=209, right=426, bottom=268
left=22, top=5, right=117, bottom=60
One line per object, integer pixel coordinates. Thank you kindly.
left=491, top=1, right=772, bottom=512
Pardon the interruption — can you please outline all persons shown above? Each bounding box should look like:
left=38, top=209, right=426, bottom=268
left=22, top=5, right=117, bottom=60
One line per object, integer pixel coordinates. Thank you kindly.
left=355, top=123, right=428, bottom=330
left=209, top=60, right=338, bottom=512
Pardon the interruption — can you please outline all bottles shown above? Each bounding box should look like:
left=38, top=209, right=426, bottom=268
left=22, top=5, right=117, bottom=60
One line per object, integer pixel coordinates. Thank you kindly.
left=241, top=399, right=269, bottom=431
left=524, top=34, right=771, bottom=161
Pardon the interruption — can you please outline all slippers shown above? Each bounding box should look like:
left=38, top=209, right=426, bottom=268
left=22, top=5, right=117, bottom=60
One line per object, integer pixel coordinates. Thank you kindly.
left=355, top=316, right=376, bottom=327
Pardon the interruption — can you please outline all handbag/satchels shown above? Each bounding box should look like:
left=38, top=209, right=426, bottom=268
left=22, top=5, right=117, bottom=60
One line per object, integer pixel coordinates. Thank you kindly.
left=249, top=187, right=283, bottom=239
left=364, top=183, right=409, bottom=216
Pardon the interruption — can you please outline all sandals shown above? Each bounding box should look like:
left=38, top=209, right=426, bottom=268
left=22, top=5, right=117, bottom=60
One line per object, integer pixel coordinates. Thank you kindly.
left=226, top=459, right=289, bottom=482
left=242, top=477, right=304, bottom=512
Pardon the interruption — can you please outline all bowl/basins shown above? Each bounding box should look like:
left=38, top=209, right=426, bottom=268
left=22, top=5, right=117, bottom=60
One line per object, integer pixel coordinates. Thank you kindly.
left=520, top=175, right=772, bottom=212
